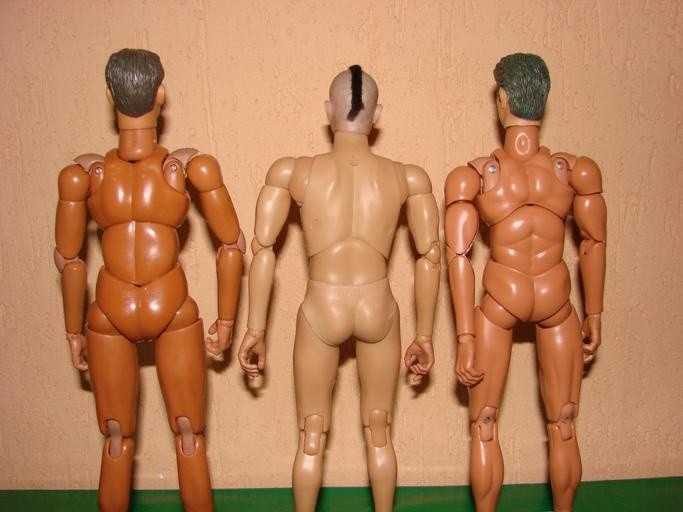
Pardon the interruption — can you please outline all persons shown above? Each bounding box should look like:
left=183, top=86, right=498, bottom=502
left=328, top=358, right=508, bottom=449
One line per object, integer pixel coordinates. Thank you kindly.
left=55, top=48, right=246, bottom=511
left=446, top=53, right=607, bottom=512
left=240, top=64, right=441, bottom=511
